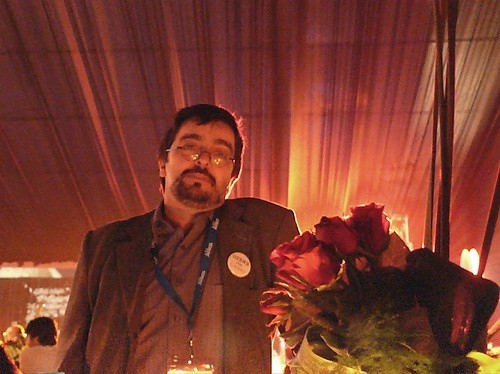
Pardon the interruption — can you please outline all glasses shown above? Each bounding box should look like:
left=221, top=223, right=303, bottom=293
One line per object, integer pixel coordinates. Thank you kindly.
left=164, top=143, right=236, bottom=168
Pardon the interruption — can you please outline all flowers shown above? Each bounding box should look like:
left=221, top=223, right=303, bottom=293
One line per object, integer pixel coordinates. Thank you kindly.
left=2, top=322, right=26, bottom=372
left=260, top=202, right=500, bottom=374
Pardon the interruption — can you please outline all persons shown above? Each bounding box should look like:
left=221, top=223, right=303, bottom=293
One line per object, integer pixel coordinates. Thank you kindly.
left=53, top=104, right=303, bottom=374
left=20, top=316, right=57, bottom=374
